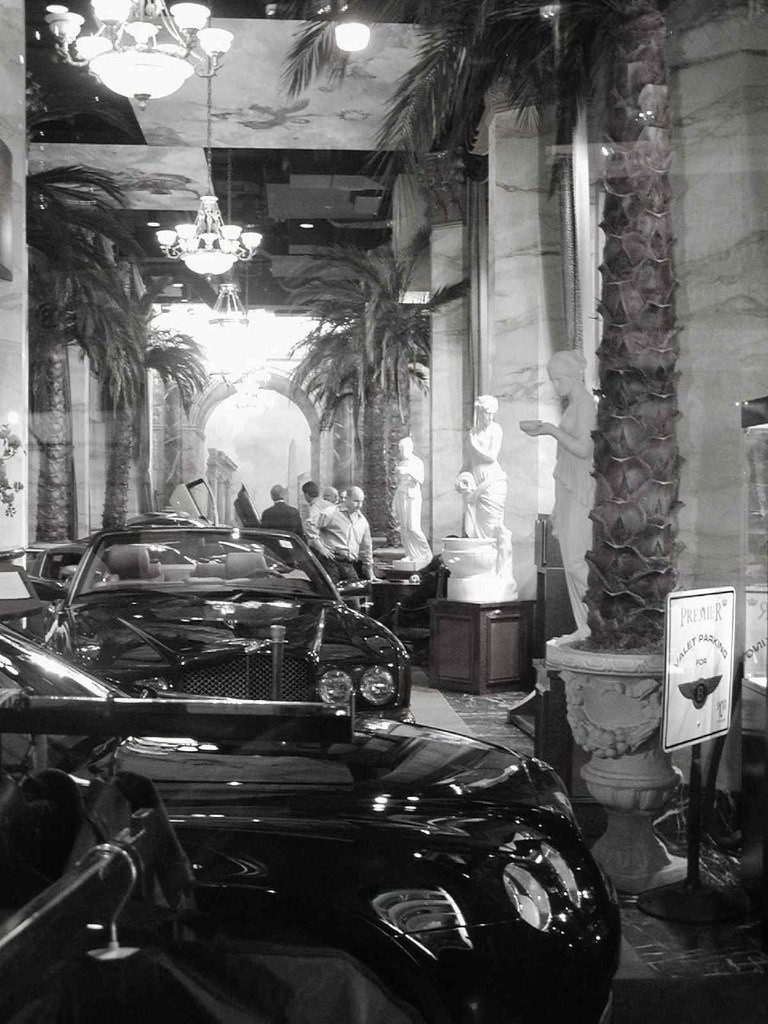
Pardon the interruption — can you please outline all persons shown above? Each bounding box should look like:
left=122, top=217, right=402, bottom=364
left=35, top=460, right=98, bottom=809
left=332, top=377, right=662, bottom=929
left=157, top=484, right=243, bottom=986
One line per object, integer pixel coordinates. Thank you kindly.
left=524, top=348, right=599, bottom=643
left=455, top=395, right=508, bottom=539
left=305, top=486, right=377, bottom=610
left=302, top=481, right=347, bottom=516
left=261, top=484, right=304, bottom=537
left=392, top=436, right=433, bottom=562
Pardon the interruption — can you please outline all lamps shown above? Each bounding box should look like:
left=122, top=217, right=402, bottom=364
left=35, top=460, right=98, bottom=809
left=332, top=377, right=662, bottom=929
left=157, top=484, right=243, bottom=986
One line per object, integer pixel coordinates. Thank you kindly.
left=43, top=0, right=233, bottom=99
left=207, top=149, right=249, bottom=337
left=154, top=0, right=264, bottom=277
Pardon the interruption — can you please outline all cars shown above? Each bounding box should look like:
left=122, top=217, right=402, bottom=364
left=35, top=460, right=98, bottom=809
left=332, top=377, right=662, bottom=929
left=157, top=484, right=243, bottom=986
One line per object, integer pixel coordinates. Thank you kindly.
left=0, top=479, right=623, bottom=1024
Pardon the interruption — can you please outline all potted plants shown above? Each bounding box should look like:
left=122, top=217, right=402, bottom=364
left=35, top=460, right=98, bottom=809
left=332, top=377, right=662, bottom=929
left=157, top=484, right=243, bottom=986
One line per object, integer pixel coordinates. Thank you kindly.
left=544, top=1, right=686, bottom=894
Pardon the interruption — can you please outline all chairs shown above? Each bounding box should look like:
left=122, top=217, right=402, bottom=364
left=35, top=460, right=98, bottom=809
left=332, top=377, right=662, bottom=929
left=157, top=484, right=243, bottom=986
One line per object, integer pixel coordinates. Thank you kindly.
left=149, top=562, right=165, bottom=581
left=103, top=545, right=150, bottom=581
left=226, top=552, right=267, bottom=579
left=190, top=563, right=225, bottom=578
